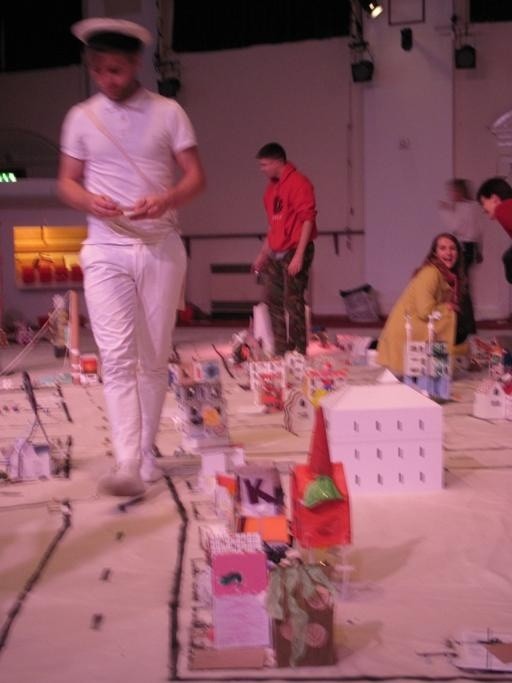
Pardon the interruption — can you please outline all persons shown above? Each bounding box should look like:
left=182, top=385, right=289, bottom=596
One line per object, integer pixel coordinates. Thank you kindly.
left=54, top=11, right=211, bottom=500
left=476, top=176, right=512, bottom=284
left=438, top=175, right=487, bottom=346
left=249, top=140, right=320, bottom=359
left=370, top=231, right=468, bottom=377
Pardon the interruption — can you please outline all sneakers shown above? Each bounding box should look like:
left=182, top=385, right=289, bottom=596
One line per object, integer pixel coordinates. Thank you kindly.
left=139, top=452, right=163, bottom=485
left=101, top=459, right=145, bottom=496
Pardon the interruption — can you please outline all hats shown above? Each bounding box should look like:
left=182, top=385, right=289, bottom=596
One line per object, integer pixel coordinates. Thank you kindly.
left=73, top=15, right=153, bottom=49
